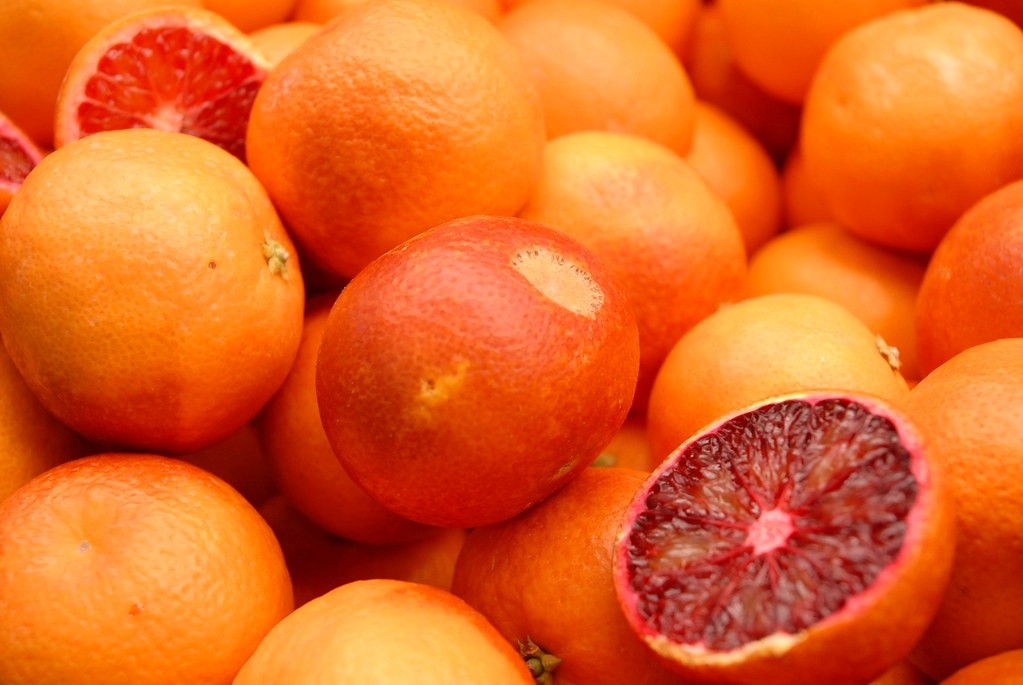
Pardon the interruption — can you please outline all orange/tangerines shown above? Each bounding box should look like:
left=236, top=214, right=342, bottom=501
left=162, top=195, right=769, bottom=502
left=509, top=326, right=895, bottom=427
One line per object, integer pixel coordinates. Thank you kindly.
left=0, top=0, right=1023, bottom=685
